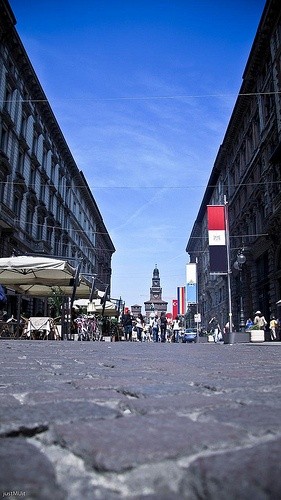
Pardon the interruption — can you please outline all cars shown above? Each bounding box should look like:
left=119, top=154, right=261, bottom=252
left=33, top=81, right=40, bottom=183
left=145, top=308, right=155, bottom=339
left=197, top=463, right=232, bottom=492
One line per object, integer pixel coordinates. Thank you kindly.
left=120, top=320, right=199, bottom=344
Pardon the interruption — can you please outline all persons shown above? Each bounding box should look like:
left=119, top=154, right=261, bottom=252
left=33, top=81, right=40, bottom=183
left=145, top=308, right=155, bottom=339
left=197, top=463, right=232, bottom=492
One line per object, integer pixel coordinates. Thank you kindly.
left=76, top=311, right=281, bottom=343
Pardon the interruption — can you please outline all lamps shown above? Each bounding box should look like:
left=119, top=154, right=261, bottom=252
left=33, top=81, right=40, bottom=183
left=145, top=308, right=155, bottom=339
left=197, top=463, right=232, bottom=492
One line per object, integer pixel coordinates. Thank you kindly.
left=232, top=248, right=247, bottom=271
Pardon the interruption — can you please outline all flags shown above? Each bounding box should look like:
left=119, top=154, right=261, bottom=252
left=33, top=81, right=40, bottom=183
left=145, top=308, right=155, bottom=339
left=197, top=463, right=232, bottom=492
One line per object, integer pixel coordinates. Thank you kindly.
left=177, top=287, right=186, bottom=314
left=185, top=264, right=196, bottom=284
left=172, top=299, right=178, bottom=320
left=207, top=206, right=228, bottom=273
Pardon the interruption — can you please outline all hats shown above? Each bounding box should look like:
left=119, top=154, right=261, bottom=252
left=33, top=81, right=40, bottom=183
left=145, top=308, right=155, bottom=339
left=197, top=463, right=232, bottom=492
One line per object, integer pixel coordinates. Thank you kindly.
left=255, top=311, right=262, bottom=314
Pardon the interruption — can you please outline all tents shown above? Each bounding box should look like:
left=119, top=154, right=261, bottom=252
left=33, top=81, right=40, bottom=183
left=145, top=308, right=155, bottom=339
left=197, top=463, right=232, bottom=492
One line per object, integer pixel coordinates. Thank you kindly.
left=0, top=254, right=121, bottom=341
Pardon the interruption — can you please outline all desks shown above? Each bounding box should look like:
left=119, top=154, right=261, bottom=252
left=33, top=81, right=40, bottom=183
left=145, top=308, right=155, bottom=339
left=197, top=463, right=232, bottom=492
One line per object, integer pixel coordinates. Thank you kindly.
left=0, top=315, right=67, bottom=340
